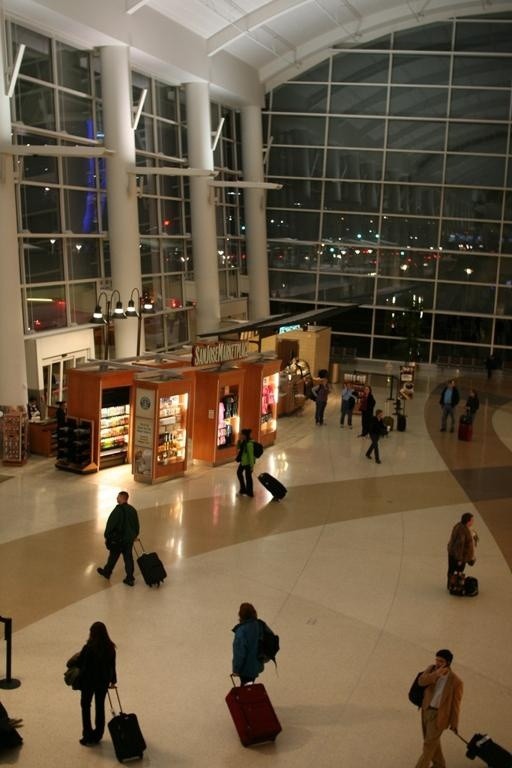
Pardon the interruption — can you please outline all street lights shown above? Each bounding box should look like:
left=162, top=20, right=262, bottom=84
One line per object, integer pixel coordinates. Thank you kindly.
left=86, top=288, right=126, bottom=360
left=125, top=284, right=154, bottom=358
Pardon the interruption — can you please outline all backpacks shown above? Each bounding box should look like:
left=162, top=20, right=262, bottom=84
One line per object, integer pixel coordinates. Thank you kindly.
left=254, top=617, right=282, bottom=673
left=253, top=441, right=264, bottom=458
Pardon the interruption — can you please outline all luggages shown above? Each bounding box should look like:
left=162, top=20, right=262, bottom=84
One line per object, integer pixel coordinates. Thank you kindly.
left=457, top=406, right=474, bottom=442
left=132, top=536, right=168, bottom=589
left=223, top=672, right=285, bottom=747
left=396, top=400, right=408, bottom=432
left=104, top=683, right=147, bottom=764
left=449, top=725, right=512, bottom=768
left=383, top=399, right=395, bottom=432
left=250, top=467, right=288, bottom=502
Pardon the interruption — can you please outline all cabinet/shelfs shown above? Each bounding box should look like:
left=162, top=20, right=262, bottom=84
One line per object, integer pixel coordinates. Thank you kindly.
left=0, top=336, right=316, bottom=486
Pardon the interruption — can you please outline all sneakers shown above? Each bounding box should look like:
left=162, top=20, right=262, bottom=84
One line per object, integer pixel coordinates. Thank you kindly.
left=95, top=566, right=112, bottom=580
left=121, top=575, right=137, bottom=586
left=374, top=458, right=382, bottom=463
left=364, top=451, right=372, bottom=459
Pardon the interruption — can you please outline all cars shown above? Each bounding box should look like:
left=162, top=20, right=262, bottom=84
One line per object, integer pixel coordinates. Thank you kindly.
left=27, top=214, right=489, bottom=346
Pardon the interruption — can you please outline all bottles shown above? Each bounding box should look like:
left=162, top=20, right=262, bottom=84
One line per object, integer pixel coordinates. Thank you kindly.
left=101, top=405, right=128, bottom=449
left=160, top=401, right=183, bottom=464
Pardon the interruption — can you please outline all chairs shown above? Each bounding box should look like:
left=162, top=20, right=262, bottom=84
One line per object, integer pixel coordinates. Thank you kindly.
left=435, top=354, right=511, bottom=378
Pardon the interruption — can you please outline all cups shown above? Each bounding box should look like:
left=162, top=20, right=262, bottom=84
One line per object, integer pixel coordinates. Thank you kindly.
left=403, top=383, right=413, bottom=394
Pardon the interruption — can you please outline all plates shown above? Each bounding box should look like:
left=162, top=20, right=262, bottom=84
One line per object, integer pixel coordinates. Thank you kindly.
left=399, top=388, right=412, bottom=399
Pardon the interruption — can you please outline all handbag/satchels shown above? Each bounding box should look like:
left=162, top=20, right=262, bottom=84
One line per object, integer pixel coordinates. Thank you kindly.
left=448, top=564, right=479, bottom=597
left=407, top=664, right=436, bottom=711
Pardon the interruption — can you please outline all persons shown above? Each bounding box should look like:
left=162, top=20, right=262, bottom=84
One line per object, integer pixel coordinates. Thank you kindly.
left=366, top=409, right=381, bottom=463
left=464, top=388, right=480, bottom=423
left=341, top=383, right=356, bottom=429
left=447, top=512, right=477, bottom=589
left=237, top=428, right=255, bottom=497
left=97, top=491, right=139, bottom=586
left=69, top=621, right=118, bottom=747
left=359, top=385, right=376, bottom=437
left=416, top=649, right=464, bottom=766
left=312, top=377, right=329, bottom=426
left=55, top=401, right=67, bottom=459
left=231, top=602, right=264, bottom=688
left=439, top=379, right=460, bottom=433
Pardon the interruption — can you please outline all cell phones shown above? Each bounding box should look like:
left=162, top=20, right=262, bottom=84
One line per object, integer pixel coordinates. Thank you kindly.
left=443, top=664, right=450, bottom=668
